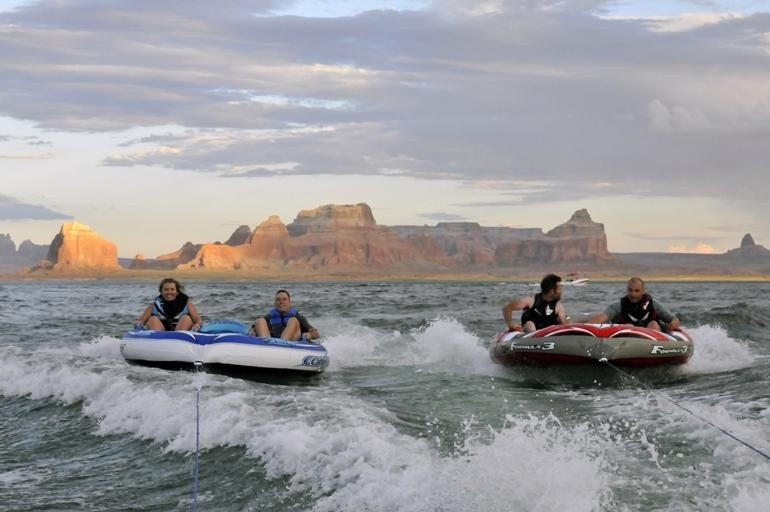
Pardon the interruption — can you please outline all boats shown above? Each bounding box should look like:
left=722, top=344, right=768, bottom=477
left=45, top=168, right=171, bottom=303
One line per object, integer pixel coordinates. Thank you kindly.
left=121, top=320, right=329, bottom=380
left=489, top=321, right=695, bottom=368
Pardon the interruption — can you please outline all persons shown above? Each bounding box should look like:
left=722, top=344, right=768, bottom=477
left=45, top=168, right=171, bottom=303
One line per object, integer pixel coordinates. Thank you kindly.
left=248, top=290, right=320, bottom=340
left=503, top=273, right=568, bottom=334
left=134, top=278, right=203, bottom=332
left=588, top=277, right=680, bottom=333
